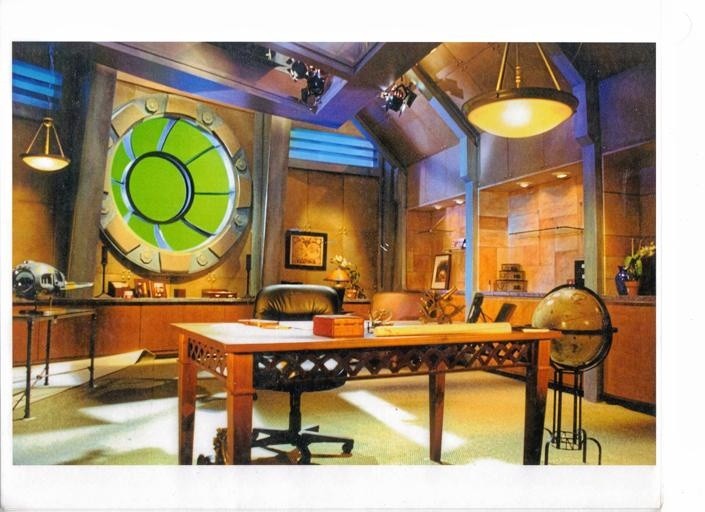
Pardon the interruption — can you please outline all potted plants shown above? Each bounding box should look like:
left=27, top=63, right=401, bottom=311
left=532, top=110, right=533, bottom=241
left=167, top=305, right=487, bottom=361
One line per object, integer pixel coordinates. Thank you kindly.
left=330, top=254, right=360, bottom=300
left=623, top=253, right=643, bottom=295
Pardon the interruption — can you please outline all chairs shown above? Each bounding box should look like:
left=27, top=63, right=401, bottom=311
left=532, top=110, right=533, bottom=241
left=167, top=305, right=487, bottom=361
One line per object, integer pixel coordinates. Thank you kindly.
left=250, top=286, right=356, bottom=465
left=354, top=290, right=432, bottom=367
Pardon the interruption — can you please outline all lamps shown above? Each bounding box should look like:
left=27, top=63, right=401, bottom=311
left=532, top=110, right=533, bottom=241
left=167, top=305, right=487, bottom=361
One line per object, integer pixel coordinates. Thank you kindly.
left=284, top=57, right=329, bottom=109
left=323, top=267, right=352, bottom=288
left=460, top=44, right=579, bottom=139
left=385, top=82, right=419, bottom=118
left=18, top=44, right=73, bottom=173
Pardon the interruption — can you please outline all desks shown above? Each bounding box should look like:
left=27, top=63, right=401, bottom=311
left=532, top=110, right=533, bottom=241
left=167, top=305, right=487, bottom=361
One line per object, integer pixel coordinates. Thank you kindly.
left=14, top=306, right=98, bottom=419
left=169, top=320, right=562, bottom=465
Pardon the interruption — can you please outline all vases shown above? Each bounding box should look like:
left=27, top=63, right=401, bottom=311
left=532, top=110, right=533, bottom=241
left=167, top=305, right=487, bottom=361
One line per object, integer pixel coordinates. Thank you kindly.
left=616, top=265, right=628, bottom=295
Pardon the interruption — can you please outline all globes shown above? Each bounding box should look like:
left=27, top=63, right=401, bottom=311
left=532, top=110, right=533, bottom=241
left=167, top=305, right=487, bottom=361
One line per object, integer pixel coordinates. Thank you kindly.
left=525, top=283, right=618, bottom=465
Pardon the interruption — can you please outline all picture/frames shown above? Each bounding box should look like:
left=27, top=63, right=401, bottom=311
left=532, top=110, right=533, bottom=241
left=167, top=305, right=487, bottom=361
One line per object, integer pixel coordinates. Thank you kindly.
left=430, top=253, right=452, bottom=290
left=285, top=229, right=328, bottom=272
left=134, top=278, right=167, bottom=299
